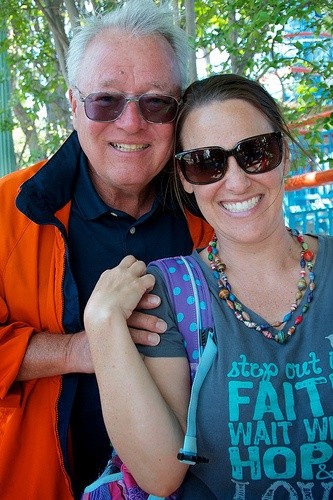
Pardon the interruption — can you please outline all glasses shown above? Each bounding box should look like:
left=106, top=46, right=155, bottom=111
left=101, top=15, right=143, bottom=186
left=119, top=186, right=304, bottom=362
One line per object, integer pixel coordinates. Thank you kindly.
left=75, top=92, right=182, bottom=124
left=175, top=131, right=283, bottom=186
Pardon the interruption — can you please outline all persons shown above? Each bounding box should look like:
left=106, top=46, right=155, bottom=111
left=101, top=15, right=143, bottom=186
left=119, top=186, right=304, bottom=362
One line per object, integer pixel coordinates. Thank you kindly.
left=1, top=0, right=217, bottom=499
left=83, top=73, right=333, bottom=499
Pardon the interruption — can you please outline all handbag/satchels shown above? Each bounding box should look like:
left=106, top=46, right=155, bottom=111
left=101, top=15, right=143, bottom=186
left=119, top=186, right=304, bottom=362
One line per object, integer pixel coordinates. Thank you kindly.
left=80, top=451, right=174, bottom=500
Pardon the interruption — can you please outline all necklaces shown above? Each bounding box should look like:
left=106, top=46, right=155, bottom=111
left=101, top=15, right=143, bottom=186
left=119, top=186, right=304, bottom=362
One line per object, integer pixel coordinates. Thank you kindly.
left=206, top=226, right=317, bottom=344
left=213, top=240, right=306, bottom=330
